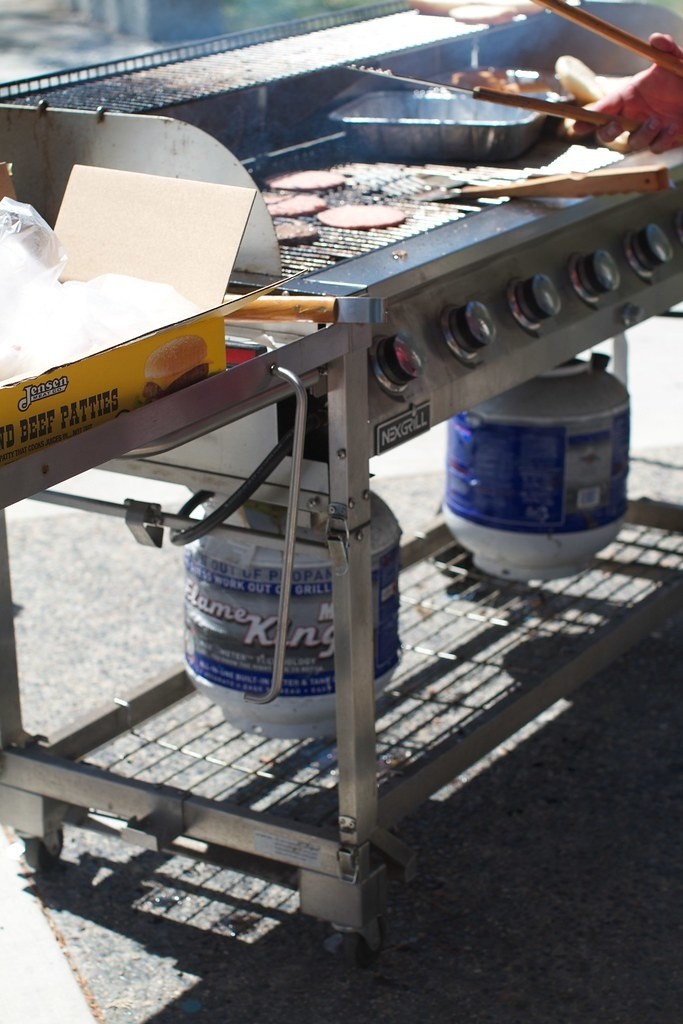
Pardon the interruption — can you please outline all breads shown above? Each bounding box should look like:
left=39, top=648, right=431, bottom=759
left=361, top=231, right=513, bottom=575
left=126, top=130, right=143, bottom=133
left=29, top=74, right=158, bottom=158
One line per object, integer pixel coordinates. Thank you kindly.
left=409, top=0, right=547, bottom=25
left=555, top=54, right=628, bottom=102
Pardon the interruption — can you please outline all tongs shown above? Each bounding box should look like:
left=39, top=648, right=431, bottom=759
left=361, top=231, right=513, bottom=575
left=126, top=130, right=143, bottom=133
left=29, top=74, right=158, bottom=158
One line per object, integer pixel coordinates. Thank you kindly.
left=340, top=1, right=683, bottom=143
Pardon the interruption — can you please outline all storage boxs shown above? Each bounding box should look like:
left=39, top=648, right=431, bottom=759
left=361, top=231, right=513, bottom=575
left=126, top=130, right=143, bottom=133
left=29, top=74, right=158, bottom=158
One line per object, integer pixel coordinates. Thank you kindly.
left=328, top=87, right=562, bottom=163
left=0, top=157, right=309, bottom=469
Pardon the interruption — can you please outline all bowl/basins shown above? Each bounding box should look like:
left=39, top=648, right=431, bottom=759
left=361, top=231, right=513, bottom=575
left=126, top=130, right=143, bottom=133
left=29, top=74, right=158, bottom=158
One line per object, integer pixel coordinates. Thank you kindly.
left=328, top=88, right=560, bottom=164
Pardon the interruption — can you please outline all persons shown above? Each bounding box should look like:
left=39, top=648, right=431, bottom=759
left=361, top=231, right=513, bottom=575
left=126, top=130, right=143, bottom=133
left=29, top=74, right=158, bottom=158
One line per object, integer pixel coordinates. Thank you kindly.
left=573, top=34, right=683, bottom=154
